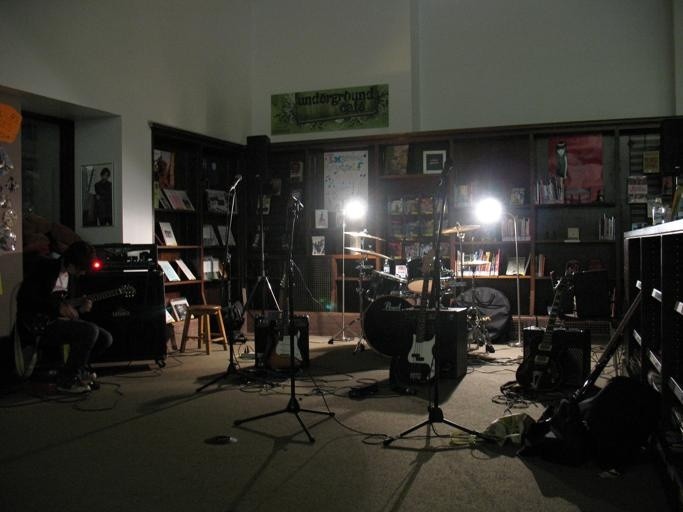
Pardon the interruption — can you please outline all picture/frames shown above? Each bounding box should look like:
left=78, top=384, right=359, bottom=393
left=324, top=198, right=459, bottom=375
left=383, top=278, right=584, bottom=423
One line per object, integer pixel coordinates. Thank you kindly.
left=81, top=162, right=114, bottom=229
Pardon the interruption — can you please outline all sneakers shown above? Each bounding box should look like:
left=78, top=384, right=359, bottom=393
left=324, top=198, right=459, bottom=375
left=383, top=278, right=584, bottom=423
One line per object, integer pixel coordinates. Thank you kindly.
left=56, top=376, right=93, bottom=394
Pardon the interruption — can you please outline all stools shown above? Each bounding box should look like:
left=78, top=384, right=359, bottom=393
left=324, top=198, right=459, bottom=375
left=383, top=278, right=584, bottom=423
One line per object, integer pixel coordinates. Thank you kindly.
left=180, top=303, right=228, bottom=356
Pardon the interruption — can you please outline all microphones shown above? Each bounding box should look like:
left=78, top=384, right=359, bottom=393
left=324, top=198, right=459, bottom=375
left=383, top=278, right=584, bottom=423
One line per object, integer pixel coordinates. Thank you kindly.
left=228, top=175, right=243, bottom=192
left=440, top=157, right=457, bottom=180
left=292, top=195, right=304, bottom=208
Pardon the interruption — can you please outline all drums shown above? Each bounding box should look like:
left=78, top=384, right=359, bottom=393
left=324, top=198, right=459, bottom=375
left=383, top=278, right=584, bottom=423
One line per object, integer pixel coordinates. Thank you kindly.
left=407, top=255, right=434, bottom=296
left=361, top=295, right=418, bottom=360
left=369, top=270, right=407, bottom=294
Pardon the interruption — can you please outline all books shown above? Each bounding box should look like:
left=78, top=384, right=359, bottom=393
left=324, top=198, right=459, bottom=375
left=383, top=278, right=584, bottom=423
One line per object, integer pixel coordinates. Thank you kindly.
left=454, top=216, right=530, bottom=276
left=534, top=176, right=564, bottom=205
left=151, top=181, right=159, bottom=208
left=596, top=213, right=615, bottom=240
left=510, top=187, right=525, bottom=206
left=384, top=196, right=450, bottom=258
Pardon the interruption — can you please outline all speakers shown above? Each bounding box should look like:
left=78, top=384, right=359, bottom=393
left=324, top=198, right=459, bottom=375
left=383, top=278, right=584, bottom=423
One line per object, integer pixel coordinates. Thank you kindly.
left=385, top=306, right=468, bottom=379
left=521, top=326, right=591, bottom=391
left=247, top=136, right=274, bottom=184
left=70, top=270, right=168, bottom=369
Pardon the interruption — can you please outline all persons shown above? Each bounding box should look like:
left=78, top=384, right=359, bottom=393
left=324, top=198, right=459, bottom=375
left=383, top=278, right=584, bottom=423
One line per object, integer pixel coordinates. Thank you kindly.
left=94, top=168, right=112, bottom=225
left=13, top=241, right=112, bottom=394
left=554, top=140, right=568, bottom=179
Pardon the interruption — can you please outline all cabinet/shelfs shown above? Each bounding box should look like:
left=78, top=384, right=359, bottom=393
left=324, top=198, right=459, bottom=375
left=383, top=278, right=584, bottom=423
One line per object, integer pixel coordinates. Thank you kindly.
left=370, top=114, right=683, bottom=341
left=146, top=119, right=256, bottom=348
left=621, top=220, right=683, bottom=478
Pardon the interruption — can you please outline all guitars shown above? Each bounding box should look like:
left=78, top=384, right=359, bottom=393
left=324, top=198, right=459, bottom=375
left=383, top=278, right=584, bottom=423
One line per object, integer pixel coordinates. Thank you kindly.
left=17, top=283, right=137, bottom=334
left=515, top=276, right=570, bottom=394
left=263, top=244, right=305, bottom=375
left=407, top=249, right=439, bottom=384
left=81, top=166, right=97, bottom=227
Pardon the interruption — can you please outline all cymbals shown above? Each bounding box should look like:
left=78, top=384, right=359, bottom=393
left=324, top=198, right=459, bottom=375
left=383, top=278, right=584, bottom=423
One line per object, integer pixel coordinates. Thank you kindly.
left=343, top=231, right=387, bottom=242
left=343, top=246, right=393, bottom=262
left=461, top=261, right=488, bottom=265
left=438, top=224, right=482, bottom=235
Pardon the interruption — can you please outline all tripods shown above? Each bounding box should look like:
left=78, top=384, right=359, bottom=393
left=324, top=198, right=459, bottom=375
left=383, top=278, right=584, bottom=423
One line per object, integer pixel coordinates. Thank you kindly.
left=241, top=184, right=284, bottom=320
left=382, top=180, right=499, bottom=448
left=233, top=204, right=335, bottom=444
left=195, top=192, right=277, bottom=394
left=328, top=255, right=366, bottom=355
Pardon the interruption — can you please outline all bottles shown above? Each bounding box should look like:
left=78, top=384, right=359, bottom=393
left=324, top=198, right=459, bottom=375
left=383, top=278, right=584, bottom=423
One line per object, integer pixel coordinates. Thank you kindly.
left=383, top=258, right=390, bottom=274
left=652, top=198, right=665, bottom=226
